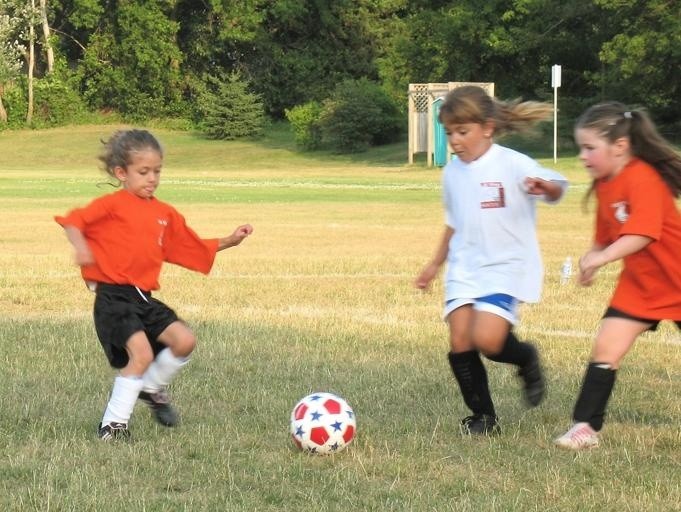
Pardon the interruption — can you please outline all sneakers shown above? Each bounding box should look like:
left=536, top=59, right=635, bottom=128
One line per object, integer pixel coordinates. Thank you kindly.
left=135, top=388, right=180, bottom=429
left=552, top=421, right=601, bottom=452
left=98, top=420, right=133, bottom=447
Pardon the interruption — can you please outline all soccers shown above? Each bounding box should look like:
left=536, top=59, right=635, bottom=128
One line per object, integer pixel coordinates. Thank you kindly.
left=290, top=392, right=357, bottom=455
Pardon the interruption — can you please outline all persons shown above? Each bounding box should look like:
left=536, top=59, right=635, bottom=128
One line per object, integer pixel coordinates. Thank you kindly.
left=555, top=102, right=681, bottom=448
left=52, top=128, right=253, bottom=443
left=414, top=85, right=570, bottom=440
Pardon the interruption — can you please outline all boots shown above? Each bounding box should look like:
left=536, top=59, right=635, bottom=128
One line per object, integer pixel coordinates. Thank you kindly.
left=445, top=347, right=504, bottom=437
left=483, top=331, right=549, bottom=408
